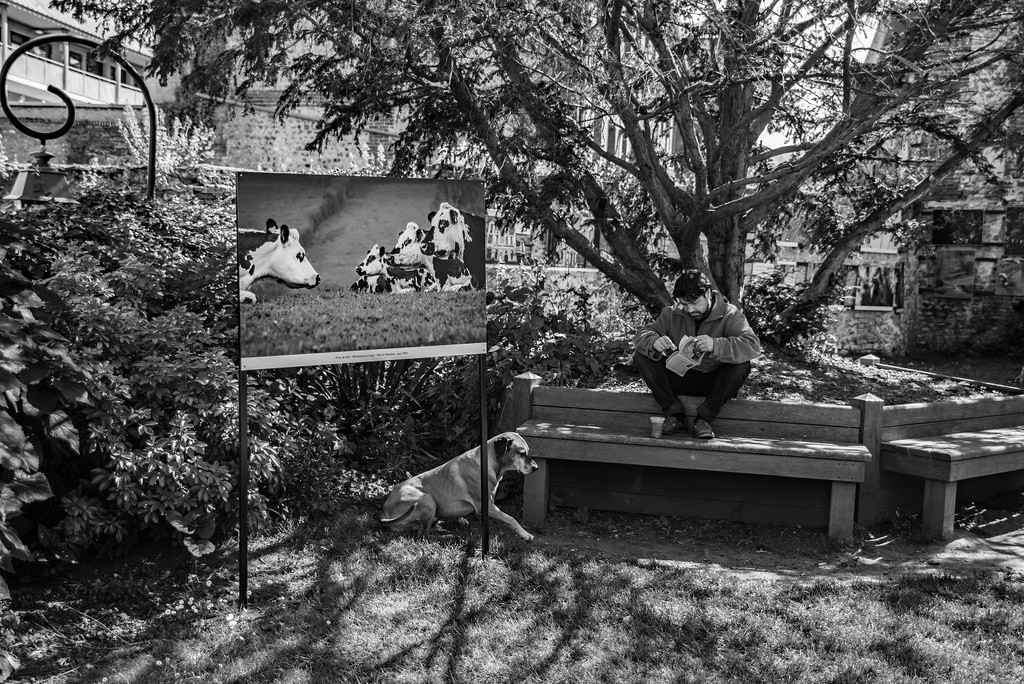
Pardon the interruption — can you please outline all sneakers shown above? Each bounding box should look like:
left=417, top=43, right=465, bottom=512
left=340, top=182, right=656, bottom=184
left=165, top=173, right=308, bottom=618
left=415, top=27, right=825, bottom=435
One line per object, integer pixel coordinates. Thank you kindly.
left=693, top=418, right=715, bottom=438
left=661, top=416, right=689, bottom=434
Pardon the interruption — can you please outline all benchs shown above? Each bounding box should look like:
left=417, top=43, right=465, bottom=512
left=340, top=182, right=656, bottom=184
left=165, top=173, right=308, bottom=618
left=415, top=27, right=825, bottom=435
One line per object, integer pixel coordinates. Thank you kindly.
left=880, top=425, right=1024, bottom=540
left=515, top=418, right=871, bottom=545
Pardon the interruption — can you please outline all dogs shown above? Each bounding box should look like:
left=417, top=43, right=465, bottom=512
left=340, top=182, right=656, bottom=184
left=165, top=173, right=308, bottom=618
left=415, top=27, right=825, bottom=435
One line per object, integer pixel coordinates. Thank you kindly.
left=379, top=430, right=539, bottom=544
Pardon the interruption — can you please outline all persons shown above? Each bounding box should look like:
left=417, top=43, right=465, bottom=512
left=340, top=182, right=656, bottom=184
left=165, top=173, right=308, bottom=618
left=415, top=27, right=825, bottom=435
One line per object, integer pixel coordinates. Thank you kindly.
left=632, top=268, right=761, bottom=439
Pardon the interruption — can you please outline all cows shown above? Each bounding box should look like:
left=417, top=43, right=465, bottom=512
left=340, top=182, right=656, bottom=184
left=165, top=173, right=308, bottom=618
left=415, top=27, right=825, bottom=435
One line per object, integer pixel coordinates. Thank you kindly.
left=239, top=217, right=321, bottom=306
left=388, top=222, right=440, bottom=293
left=350, top=243, right=393, bottom=295
left=420, top=202, right=486, bottom=290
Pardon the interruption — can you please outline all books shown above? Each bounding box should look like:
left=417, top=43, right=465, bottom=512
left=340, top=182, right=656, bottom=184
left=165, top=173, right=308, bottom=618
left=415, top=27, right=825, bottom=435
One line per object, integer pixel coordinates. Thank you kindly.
left=665, top=334, right=707, bottom=377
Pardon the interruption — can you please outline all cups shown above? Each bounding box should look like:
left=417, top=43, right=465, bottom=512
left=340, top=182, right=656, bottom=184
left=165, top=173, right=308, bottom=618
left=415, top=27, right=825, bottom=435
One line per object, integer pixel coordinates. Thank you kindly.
left=649, top=417, right=665, bottom=439
